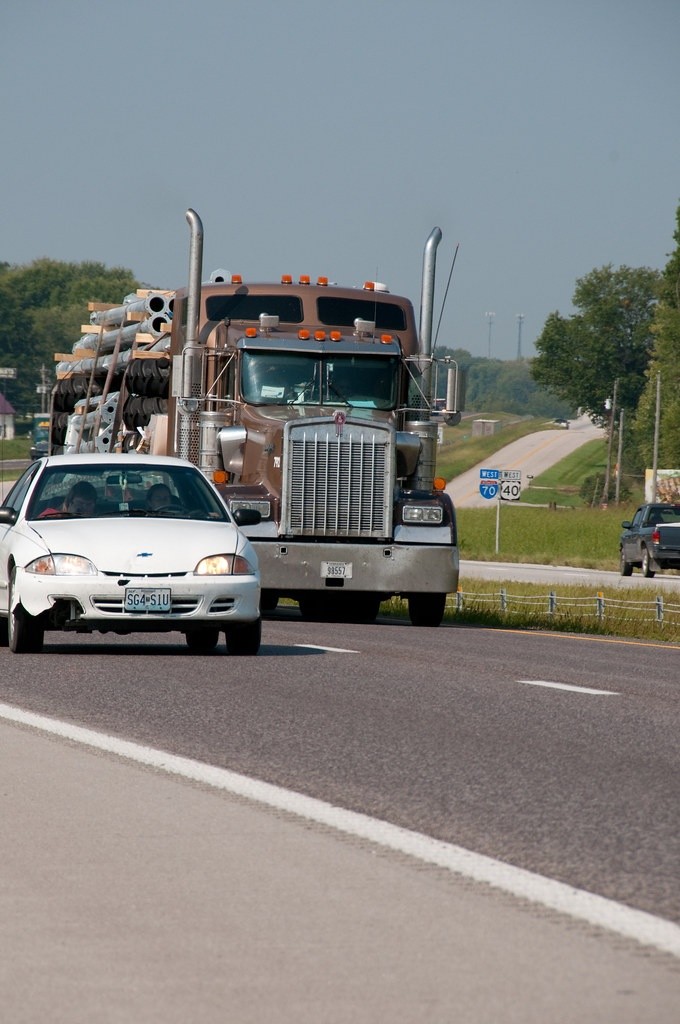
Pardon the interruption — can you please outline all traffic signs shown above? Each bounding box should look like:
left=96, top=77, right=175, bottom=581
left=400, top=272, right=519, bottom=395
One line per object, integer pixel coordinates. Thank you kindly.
left=501, top=470, right=521, bottom=480
left=480, top=469, right=498, bottom=479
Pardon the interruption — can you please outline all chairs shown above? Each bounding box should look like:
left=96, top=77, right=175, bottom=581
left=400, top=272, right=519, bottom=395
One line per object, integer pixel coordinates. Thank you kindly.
left=168, top=496, right=182, bottom=512
left=651, top=516, right=664, bottom=523
left=47, top=497, right=65, bottom=509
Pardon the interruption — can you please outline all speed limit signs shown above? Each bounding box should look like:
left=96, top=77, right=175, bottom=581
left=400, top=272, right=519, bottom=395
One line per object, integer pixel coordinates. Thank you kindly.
left=500, top=481, right=521, bottom=500
left=479, top=480, right=498, bottom=499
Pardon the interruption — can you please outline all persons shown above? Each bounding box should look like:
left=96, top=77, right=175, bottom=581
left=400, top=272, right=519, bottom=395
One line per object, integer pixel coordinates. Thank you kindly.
left=651, top=509, right=663, bottom=523
left=37, top=481, right=96, bottom=519
left=145, top=483, right=221, bottom=518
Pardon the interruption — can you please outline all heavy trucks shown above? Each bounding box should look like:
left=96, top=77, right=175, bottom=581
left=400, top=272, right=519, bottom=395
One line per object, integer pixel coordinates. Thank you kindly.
left=49, top=208, right=461, bottom=626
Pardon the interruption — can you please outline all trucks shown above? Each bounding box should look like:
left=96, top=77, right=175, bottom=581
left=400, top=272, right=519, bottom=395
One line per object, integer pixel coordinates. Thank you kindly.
left=27, top=413, right=51, bottom=444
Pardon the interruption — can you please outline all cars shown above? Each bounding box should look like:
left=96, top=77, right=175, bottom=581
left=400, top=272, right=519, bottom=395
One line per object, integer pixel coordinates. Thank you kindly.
left=0, top=453, right=261, bottom=657
left=30, top=441, right=49, bottom=460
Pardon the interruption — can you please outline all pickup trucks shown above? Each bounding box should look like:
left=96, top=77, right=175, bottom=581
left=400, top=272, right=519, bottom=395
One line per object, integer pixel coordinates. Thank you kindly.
left=552, top=419, right=570, bottom=429
left=618, top=503, right=680, bottom=579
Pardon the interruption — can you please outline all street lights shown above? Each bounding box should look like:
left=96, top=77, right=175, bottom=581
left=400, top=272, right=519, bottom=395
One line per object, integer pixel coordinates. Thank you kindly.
left=37, top=386, right=47, bottom=413
left=604, top=398, right=615, bottom=501
left=486, top=312, right=495, bottom=359
